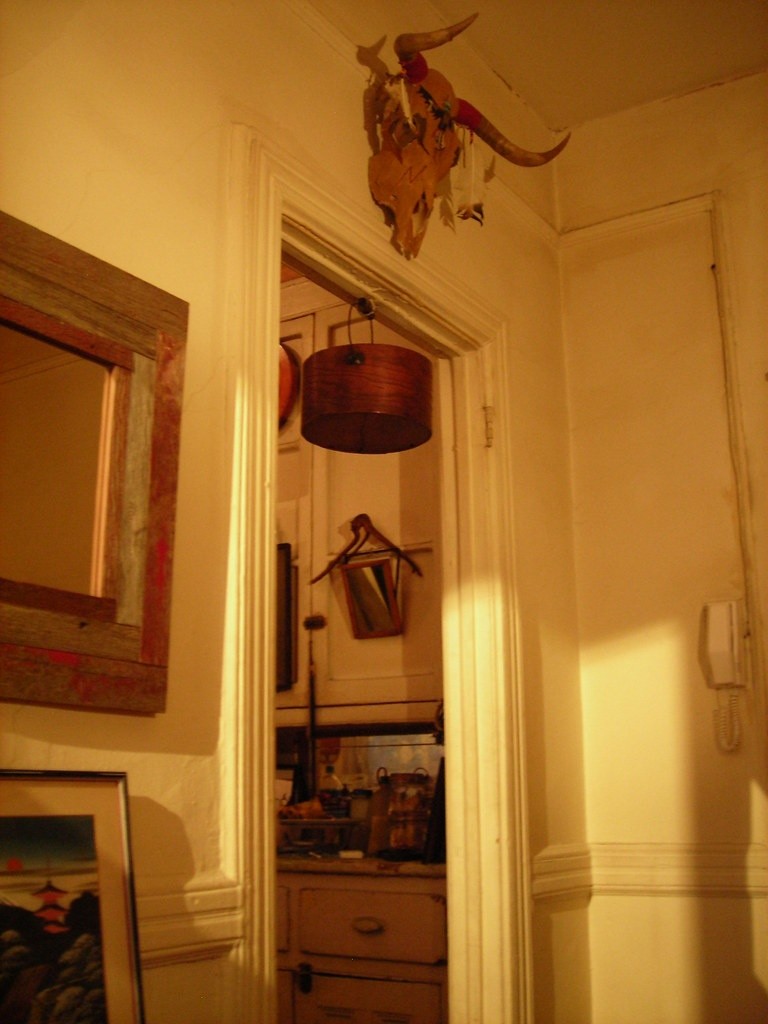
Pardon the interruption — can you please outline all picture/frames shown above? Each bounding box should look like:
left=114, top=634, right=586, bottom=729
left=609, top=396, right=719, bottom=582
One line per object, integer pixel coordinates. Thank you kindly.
left=0, top=769, right=147, bottom=1024
left=275, top=873, right=448, bottom=1024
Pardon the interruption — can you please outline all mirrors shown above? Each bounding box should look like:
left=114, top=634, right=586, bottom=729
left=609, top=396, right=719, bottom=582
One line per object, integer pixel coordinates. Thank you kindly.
left=340, top=559, right=402, bottom=639
